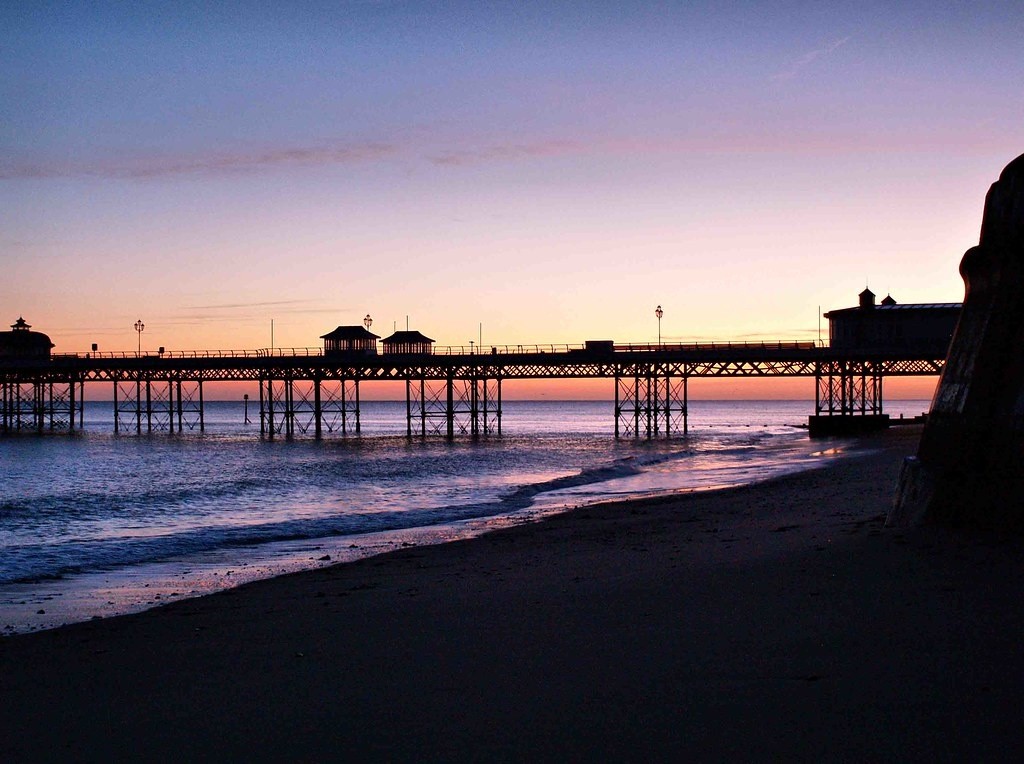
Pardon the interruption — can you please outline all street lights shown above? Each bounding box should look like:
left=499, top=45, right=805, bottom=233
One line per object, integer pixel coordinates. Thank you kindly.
left=364, top=314, right=373, bottom=332
left=134, top=319, right=145, bottom=358
left=655, top=305, right=664, bottom=351
